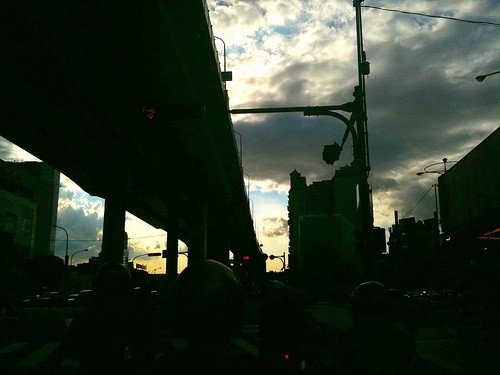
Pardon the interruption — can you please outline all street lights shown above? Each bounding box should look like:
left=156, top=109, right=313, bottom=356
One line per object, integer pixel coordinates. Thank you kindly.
left=46, top=224, right=69, bottom=266
left=70, top=249, right=89, bottom=265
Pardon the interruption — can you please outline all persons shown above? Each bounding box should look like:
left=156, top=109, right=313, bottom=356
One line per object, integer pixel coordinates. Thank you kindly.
left=61, top=258, right=416, bottom=374
